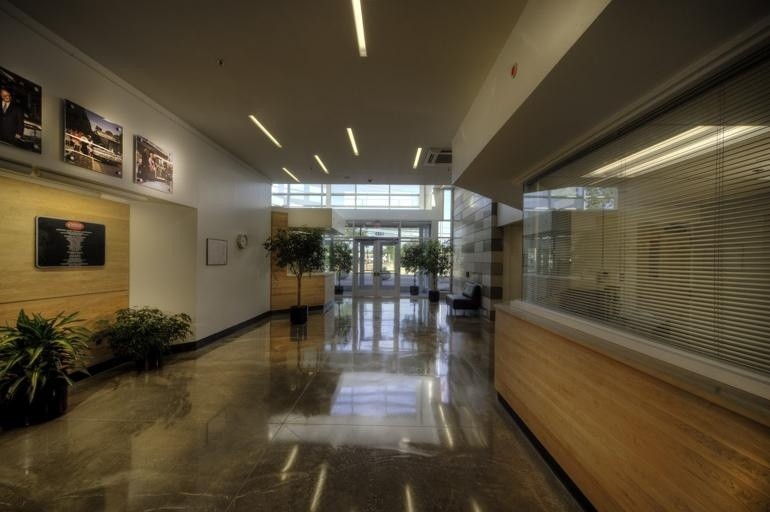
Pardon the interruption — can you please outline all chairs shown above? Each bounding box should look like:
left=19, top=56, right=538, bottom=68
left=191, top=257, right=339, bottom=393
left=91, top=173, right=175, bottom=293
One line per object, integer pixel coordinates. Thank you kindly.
left=446, top=282, right=482, bottom=318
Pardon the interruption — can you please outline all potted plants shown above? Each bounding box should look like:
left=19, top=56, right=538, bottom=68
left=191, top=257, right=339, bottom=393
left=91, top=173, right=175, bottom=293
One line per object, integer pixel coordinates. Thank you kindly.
left=400, top=238, right=455, bottom=303
left=262, top=224, right=354, bottom=325
left=1, top=305, right=194, bottom=432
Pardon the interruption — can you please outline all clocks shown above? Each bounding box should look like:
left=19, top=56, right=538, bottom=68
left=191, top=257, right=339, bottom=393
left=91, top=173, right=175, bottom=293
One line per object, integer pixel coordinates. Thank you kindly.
left=237, top=232, right=248, bottom=250
